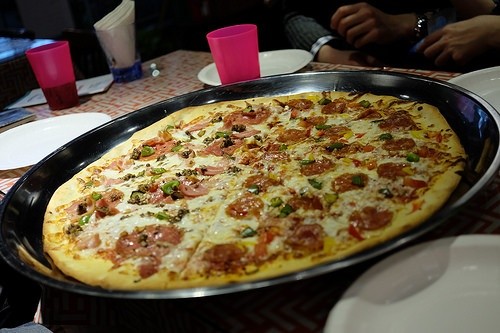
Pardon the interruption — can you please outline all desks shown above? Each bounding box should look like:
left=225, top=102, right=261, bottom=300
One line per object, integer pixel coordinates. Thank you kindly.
left=0, top=37, right=79, bottom=107
left=0, top=50, right=500, bottom=333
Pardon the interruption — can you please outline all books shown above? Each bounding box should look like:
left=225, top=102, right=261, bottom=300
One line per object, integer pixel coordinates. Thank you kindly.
left=0, top=108, right=37, bottom=134
left=2, top=73, right=114, bottom=110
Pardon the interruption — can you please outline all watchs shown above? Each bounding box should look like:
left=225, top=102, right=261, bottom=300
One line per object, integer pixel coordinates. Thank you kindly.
left=408, top=11, right=429, bottom=41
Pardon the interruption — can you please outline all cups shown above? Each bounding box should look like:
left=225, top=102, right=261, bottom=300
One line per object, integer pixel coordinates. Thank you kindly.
left=206, top=24, right=261, bottom=85
left=95, top=24, right=145, bottom=83
left=25, top=41, right=80, bottom=111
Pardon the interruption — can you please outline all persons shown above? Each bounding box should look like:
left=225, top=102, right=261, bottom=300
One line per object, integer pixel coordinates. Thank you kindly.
left=273, top=0, right=461, bottom=67
left=410, top=0, right=500, bottom=67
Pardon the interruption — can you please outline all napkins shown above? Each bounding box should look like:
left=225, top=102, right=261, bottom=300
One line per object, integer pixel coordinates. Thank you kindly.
left=93, top=1, right=136, bottom=67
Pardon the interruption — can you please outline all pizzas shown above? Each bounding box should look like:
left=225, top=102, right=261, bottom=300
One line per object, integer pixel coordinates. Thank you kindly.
left=41, top=90, right=467, bottom=292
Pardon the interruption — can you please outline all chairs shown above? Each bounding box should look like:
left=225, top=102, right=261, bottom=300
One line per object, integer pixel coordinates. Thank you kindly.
left=14, top=0, right=86, bottom=75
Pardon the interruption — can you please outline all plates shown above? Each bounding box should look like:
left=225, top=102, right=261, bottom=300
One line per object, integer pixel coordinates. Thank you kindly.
left=325, top=234, right=500, bottom=333
left=197, top=50, right=314, bottom=86
left=447, top=66, right=500, bottom=114
left=0, top=112, right=111, bottom=170
left=2, top=70, right=499, bottom=300
